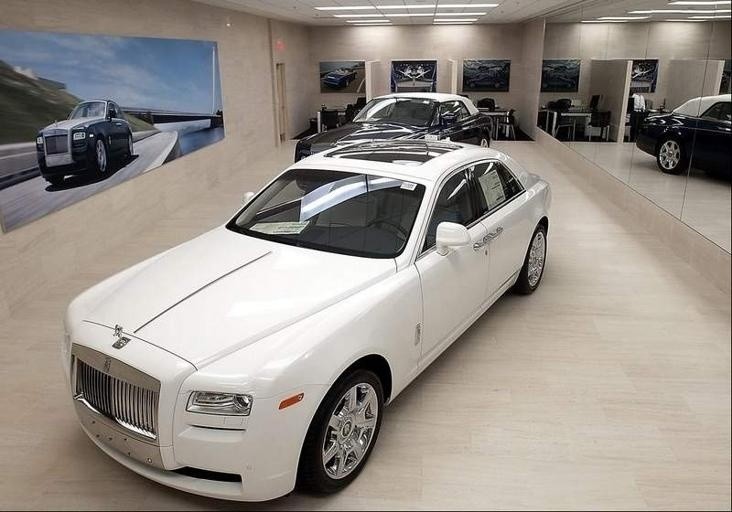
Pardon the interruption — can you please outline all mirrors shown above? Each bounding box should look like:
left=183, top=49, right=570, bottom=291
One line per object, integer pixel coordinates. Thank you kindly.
left=534, top=0, right=732, bottom=254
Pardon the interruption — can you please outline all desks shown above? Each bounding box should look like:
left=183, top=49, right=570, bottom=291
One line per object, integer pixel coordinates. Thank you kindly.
left=561, top=113, right=592, bottom=141
left=317, top=109, right=346, bottom=133
left=479, top=111, right=507, bottom=140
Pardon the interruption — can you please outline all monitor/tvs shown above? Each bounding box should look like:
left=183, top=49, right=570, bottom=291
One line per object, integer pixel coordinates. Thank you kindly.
left=627, top=98, right=634, bottom=114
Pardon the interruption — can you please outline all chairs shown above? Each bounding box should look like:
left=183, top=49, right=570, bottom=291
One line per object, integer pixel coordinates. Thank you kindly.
left=587, top=111, right=611, bottom=142
left=397, top=184, right=465, bottom=249
left=476, top=98, right=495, bottom=138
left=552, top=98, right=576, bottom=142
left=320, top=110, right=340, bottom=131
left=315, top=192, right=379, bottom=229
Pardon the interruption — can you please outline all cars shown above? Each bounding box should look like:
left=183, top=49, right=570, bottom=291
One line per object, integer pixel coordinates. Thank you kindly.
left=35, top=99, right=134, bottom=187
left=467, top=76, right=506, bottom=89
left=542, top=76, right=575, bottom=89
left=294, top=92, right=493, bottom=192
left=636, top=94, right=732, bottom=173
left=322, top=67, right=357, bottom=88
left=62, top=138, right=552, bottom=501
